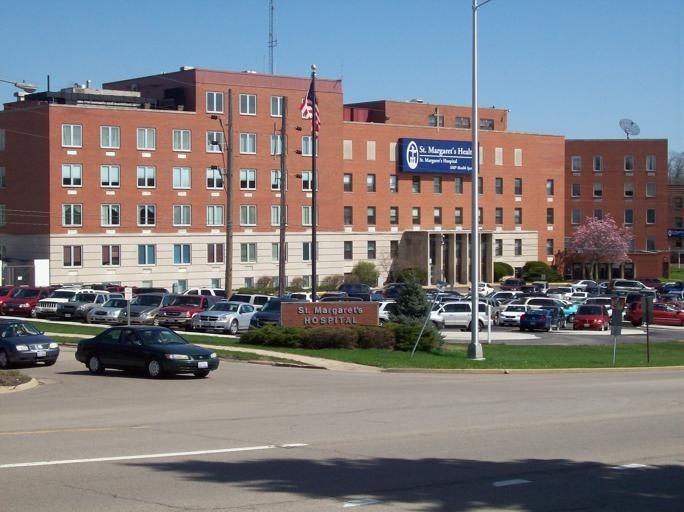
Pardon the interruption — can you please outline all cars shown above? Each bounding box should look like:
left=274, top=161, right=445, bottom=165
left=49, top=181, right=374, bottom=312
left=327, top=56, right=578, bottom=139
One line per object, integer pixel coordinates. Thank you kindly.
left=73, top=323, right=221, bottom=382
left=0, top=321, right=59, bottom=370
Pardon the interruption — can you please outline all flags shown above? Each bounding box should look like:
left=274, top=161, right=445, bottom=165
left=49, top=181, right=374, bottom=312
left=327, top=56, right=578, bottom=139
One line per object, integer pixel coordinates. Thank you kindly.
left=298, top=72, right=321, bottom=138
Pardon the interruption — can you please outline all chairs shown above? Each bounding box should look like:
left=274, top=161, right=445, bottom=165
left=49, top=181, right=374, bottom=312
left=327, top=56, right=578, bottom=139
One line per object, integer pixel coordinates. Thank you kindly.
left=106, top=333, right=161, bottom=344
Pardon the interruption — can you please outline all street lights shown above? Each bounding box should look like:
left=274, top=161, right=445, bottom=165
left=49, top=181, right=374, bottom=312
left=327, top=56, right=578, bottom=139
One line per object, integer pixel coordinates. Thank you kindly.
left=270, top=93, right=303, bottom=295
left=209, top=88, right=233, bottom=300
left=0, top=79, right=38, bottom=94
